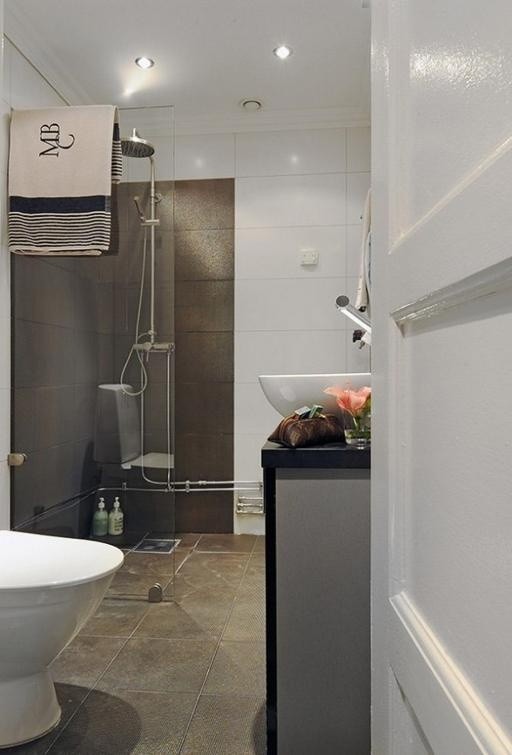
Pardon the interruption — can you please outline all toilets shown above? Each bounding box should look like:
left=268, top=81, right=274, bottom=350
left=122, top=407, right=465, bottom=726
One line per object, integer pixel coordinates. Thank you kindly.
left=1, top=529, right=125, bottom=749
left=94, top=384, right=174, bottom=531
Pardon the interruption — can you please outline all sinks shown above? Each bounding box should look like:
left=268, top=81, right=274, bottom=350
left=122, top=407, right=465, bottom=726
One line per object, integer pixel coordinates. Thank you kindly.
left=259, top=373, right=372, bottom=418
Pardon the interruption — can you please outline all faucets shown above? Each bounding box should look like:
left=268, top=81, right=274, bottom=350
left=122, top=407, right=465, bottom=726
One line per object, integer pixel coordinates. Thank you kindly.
left=334, top=296, right=372, bottom=348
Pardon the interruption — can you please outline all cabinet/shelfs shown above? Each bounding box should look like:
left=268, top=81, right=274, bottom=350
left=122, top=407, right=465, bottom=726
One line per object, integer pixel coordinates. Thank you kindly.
left=261, top=414, right=374, bottom=755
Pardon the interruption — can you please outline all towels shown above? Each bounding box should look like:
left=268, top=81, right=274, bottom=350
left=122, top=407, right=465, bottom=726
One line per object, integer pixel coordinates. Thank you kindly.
left=8, top=104, right=124, bottom=258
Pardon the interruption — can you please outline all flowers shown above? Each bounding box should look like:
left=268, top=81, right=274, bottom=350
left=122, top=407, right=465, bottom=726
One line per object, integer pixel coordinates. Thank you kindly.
left=324, top=378, right=371, bottom=414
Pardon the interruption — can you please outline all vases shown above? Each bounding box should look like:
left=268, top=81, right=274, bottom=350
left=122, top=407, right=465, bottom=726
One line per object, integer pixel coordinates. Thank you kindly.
left=337, top=416, right=371, bottom=447
